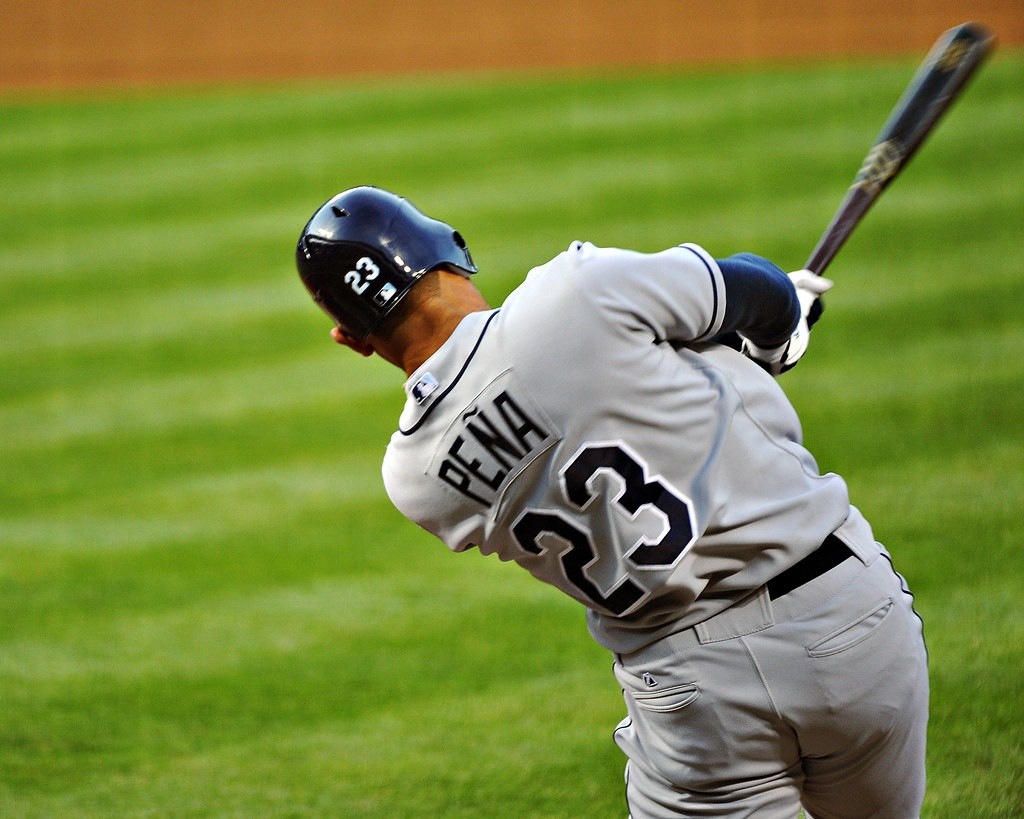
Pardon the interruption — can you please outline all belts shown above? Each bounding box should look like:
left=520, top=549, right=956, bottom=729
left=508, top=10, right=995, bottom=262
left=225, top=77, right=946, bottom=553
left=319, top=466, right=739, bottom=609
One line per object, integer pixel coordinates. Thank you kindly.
left=768, top=531, right=858, bottom=601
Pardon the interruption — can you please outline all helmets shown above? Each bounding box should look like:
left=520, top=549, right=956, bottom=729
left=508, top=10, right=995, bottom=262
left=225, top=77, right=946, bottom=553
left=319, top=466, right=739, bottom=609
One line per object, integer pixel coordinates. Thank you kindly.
left=295, top=185, right=480, bottom=343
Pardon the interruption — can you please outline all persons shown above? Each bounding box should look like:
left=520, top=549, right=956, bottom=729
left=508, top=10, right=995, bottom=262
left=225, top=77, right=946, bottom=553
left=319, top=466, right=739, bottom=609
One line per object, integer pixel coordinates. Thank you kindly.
left=293, top=186, right=931, bottom=819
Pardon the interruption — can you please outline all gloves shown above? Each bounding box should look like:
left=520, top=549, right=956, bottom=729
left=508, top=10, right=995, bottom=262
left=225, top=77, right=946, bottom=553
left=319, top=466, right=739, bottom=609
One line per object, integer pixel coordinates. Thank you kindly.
left=787, top=270, right=837, bottom=328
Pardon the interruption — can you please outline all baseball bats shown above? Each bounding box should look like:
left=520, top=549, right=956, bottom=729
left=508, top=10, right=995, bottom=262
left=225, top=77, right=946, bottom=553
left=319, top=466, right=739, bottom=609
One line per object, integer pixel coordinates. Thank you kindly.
left=803, top=20, right=999, bottom=278
